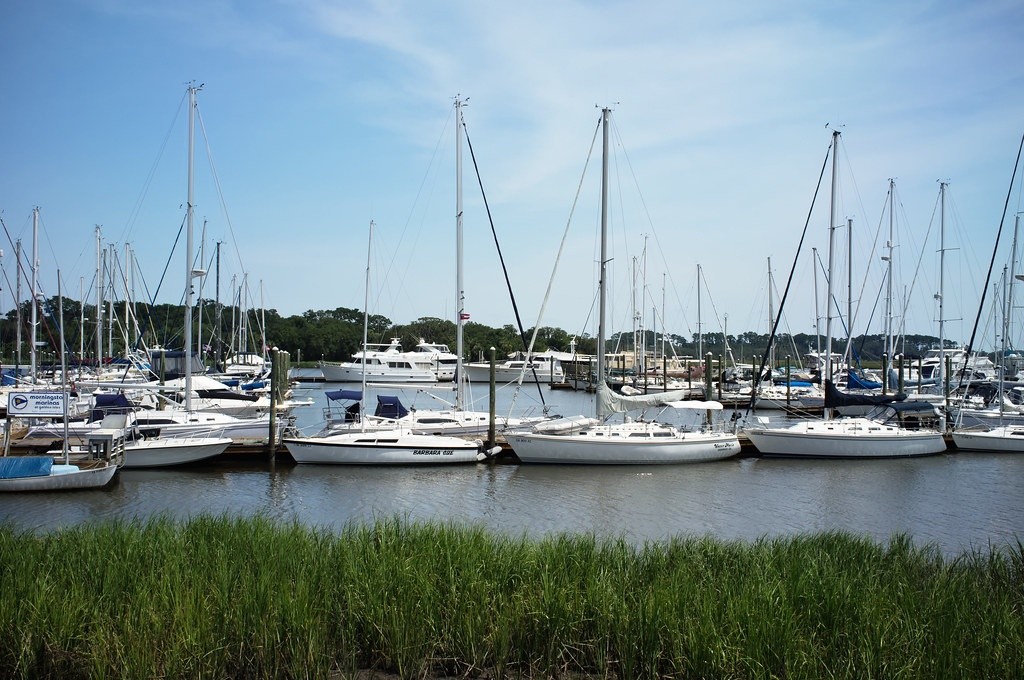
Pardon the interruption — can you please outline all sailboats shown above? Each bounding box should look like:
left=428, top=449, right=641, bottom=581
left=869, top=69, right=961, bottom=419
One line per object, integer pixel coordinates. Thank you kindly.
left=0, top=77, right=1023, bottom=491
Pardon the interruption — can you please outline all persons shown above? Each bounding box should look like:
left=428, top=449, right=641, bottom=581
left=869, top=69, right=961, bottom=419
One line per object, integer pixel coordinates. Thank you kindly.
left=52, top=383, right=83, bottom=423
left=936, top=406, right=955, bottom=431
left=722, top=371, right=727, bottom=383
